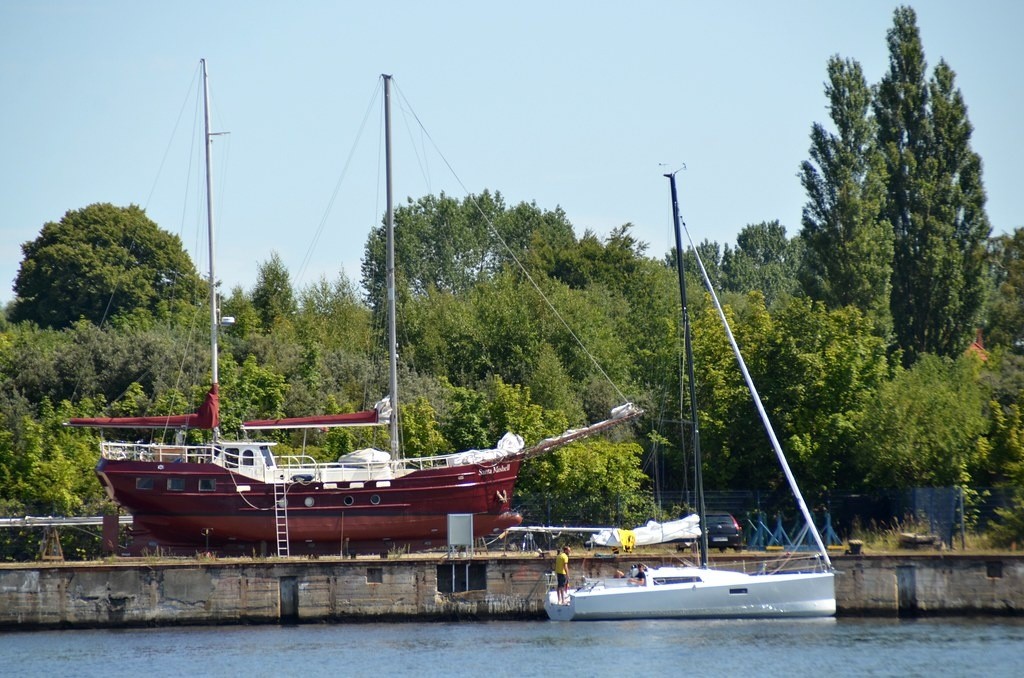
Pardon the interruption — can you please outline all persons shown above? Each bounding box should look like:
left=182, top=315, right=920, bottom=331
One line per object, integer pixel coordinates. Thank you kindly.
left=555, top=546, right=571, bottom=606
left=629, top=563, right=647, bottom=587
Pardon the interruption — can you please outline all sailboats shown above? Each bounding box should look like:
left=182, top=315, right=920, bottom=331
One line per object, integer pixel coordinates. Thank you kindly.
left=530, top=214, right=846, bottom=623
left=584, top=162, right=704, bottom=550
left=57, top=57, right=652, bottom=555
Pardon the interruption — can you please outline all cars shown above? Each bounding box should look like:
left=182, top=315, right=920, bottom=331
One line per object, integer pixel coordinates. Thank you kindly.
left=674, top=506, right=744, bottom=554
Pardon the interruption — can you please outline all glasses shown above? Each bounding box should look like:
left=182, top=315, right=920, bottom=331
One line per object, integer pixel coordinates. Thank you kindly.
left=638, top=565, right=642, bottom=568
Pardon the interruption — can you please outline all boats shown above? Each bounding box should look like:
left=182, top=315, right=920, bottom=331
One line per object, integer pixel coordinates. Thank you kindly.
left=482, top=524, right=621, bottom=552
